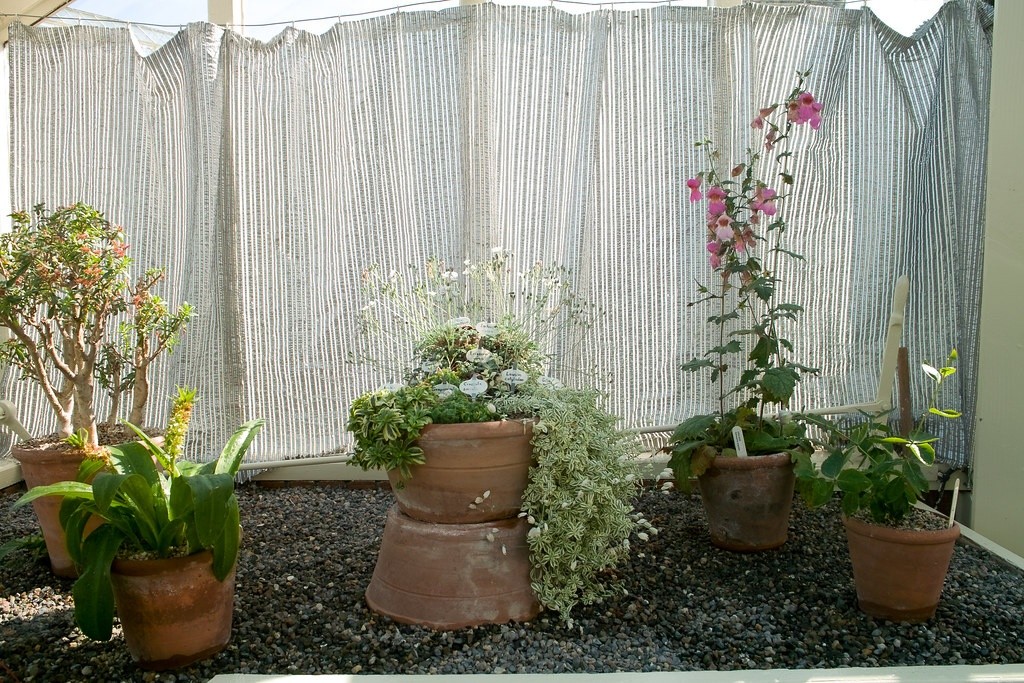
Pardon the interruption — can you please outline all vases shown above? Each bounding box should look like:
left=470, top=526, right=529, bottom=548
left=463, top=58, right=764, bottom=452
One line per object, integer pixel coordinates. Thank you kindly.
left=845, top=510, right=961, bottom=621
left=364, top=502, right=545, bottom=630
left=11, top=423, right=165, bottom=580
left=696, top=445, right=803, bottom=553
left=382, top=417, right=538, bottom=522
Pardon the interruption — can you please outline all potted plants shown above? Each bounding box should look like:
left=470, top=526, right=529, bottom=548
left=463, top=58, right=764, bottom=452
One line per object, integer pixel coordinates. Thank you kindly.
left=12, top=381, right=270, bottom=673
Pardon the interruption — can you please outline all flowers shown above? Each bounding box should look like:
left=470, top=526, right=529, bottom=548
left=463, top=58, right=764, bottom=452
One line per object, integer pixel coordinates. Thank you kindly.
left=790, top=347, right=962, bottom=523
left=664, top=65, right=836, bottom=493
left=0, top=200, right=201, bottom=451
left=346, top=247, right=676, bottom=624
